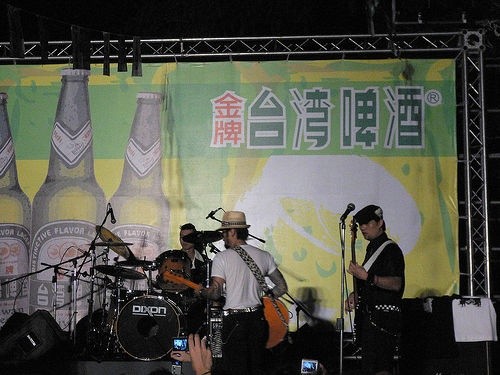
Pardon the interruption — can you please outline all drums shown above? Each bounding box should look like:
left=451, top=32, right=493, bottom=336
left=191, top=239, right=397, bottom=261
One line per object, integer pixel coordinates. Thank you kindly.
left=156, top=249, right=192, bottom=294
left=103, top=289, right=157, bottom=336
left=115, top=295, right=186, bottom=361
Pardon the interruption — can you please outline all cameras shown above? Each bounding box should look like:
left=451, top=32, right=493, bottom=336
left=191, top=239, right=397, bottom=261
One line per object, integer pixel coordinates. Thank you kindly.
left=172, top=337, right=189, bottom=351
left=300, top=358, right=318, bottom=373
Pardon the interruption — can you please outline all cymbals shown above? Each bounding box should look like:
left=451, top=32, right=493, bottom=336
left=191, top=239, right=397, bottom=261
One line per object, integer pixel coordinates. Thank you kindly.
left=95, top=224, right=131, bottom=260
left=93, top=265, right=146, bottom=279
left=116, top=259, right=153, bottom=267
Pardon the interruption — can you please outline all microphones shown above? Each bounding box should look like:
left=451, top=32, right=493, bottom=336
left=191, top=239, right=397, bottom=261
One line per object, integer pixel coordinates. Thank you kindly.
left=206, top=208, right=221, bottom=219
left=74, top=247, right=91, bottom=257
left=340, top=203, right=355, bottom=220
left=108, top=203, right=116, bottom=224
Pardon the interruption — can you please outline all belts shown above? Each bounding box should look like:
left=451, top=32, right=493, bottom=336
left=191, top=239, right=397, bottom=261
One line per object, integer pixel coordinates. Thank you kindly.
left=224, top=306, right=258, bottom=317
left=375, top=304, right=401, bottom=313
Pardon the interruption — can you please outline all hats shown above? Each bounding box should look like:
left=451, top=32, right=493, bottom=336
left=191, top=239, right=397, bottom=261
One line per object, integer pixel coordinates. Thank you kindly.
left=353, top=205, right=383, bottom=222
left=216, top=211, right=250, bottom=229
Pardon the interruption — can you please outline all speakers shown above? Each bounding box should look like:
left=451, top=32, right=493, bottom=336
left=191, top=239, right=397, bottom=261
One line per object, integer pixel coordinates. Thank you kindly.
left=0, top=309, right=174, bottom=374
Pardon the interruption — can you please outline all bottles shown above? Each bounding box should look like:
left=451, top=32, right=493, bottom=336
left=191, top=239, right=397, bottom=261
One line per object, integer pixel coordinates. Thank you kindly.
left=25, top=68, right=110, bottom=338
left=0, top=91, right=33, bottom=331
left=105, top=89, right=170, bottom=308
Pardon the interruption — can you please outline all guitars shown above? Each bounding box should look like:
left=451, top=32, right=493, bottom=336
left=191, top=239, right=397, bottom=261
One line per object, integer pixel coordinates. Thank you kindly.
left=351, top=214, right=365, bottom=346
left=161, top=269, right=290, bottom=349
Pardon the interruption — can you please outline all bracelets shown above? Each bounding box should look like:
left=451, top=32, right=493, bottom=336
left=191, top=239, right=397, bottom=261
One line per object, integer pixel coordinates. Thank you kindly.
left=367, top=273, right=374, bottom=284
left=374, top=275, right=379, bottom=285
left=201, top=371, right=211, bottom=375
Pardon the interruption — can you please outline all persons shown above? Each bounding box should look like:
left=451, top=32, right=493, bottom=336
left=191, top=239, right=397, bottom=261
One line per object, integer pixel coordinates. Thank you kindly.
left=344, top=205, right=405, bottom=375
left=156, top=223, right=213, bottom=351
left=189, top=333, right=212, bottom=375
left=194, top=211, right=288, bottom=375
